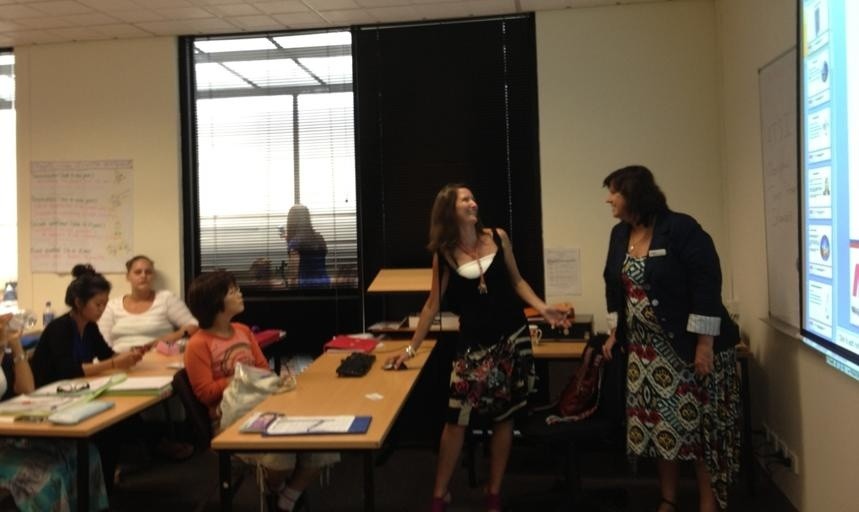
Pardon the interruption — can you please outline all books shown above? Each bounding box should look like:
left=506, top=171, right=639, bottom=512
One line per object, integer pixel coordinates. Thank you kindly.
left=107, top=377, right=174, bottom=396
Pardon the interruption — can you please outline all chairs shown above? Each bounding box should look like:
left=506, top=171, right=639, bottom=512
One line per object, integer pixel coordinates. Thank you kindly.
left=508, top=333, right=621, bottom=510
left=171, top=368, right=248, bottom=510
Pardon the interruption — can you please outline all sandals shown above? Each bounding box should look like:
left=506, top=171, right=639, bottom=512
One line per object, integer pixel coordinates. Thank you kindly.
left=482, top=485, right=502, bottom=511
left=431, top=489, right=452, bottom=511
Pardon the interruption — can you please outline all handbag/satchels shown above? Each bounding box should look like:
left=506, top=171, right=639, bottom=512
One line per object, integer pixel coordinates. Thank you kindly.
left=559, top=336, right=603, bottom=416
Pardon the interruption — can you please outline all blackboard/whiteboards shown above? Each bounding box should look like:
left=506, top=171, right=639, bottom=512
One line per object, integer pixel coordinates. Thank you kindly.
left=757, top=43, right=798, bottom=339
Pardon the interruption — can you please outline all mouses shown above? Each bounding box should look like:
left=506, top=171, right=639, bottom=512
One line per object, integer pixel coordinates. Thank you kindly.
left=382, top=356, right=406, bottom=372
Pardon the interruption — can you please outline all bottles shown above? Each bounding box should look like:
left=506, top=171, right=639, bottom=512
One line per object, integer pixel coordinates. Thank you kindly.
left=42, top=301, right=54, bottom=328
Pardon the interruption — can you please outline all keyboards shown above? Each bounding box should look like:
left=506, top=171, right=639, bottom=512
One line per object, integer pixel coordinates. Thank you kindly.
left=336, top=350, right=377, bottom=378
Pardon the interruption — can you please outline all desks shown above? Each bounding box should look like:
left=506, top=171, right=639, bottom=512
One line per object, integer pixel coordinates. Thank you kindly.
left=523, top=339, right=595, bottom=411
left=207, top=335, right=439, bottom=510
left=0, top=353, right=192, bottom=512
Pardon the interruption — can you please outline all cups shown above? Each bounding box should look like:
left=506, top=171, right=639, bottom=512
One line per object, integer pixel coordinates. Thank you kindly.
left=528, top=325, right=542, bottom=348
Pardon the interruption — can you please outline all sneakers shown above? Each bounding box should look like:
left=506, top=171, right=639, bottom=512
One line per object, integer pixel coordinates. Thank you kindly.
left=266, top=493, right=290, bottom=512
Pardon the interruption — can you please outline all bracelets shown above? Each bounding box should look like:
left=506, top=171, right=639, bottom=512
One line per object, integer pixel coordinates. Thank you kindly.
left=404, top=345, right=415, bottom=358
left=13, top=354, right=25, bottom=364
left=113, top=355, right=120, bottom=372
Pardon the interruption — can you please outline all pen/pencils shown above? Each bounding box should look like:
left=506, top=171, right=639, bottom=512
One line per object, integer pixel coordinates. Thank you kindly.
left=51, top=399, right=71, bottom=410
left=307, top=419, right=323, bottom=432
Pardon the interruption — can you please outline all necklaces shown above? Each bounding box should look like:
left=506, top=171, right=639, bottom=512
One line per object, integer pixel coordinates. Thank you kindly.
left=458, top=237, right=488, bottom=296
left=628, top=238, right=641, bottom=253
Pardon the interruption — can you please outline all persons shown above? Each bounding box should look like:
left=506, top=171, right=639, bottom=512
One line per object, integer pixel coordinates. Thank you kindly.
left=0, top=312, right=111, bottom=512
left=183, top=269, right=340, bottom=512
left=281, top=208, right=332, bottom=289
left=99, top=256, right=197, bottom=356
left=30, top=263, right=162, bottom=474
left=383, top=184, right=572, bottom=512
left=601, top=164, right=743, bottom=512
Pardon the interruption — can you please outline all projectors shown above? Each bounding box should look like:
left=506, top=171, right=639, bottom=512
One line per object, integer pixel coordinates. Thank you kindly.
left=526, top=313, right=594, bottom=343
